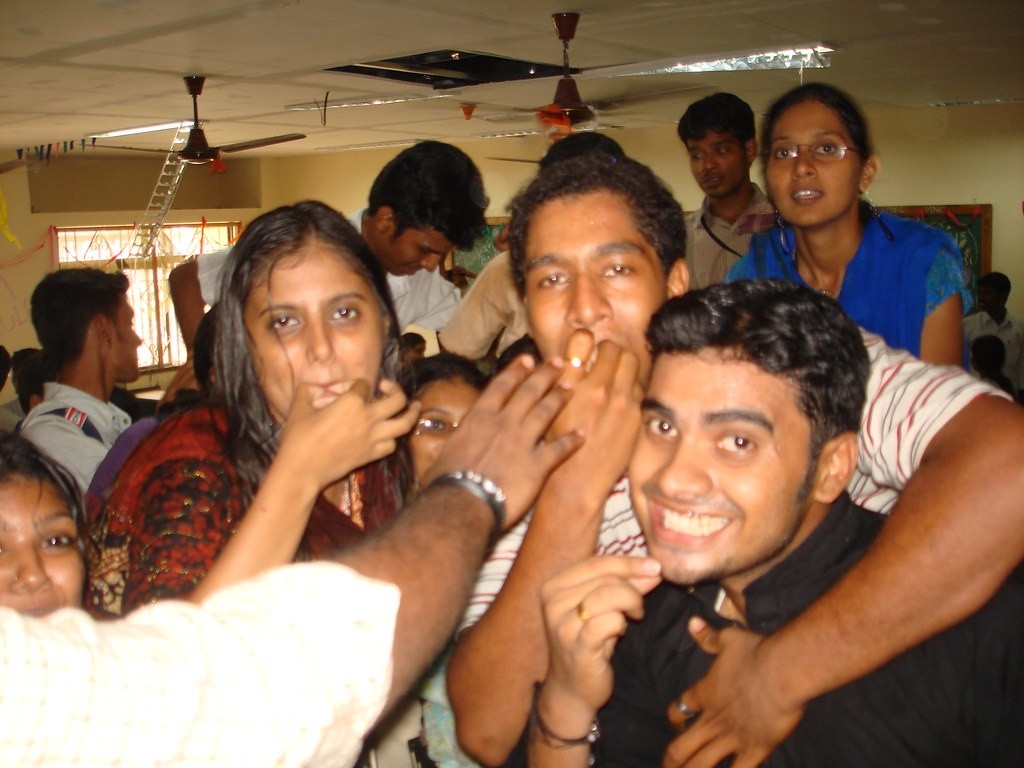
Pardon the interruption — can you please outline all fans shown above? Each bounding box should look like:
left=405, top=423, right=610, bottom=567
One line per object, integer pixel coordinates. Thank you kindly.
left=77, top=76, right=307, bottom=164
left=457, top=10, right=717, bottom=128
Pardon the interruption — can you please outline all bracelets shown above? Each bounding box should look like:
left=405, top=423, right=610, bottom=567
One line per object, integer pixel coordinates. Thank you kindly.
left=420, top=472, right=506, bottom=541
left=531, top=700, right=601, bottom=749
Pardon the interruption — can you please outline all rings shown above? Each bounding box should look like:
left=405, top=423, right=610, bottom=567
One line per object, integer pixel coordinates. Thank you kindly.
left=676, top=696, right=694, bottom=717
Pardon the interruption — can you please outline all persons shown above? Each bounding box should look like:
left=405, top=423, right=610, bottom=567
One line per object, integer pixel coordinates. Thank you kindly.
left=0, top=83, right=1024, bottom=768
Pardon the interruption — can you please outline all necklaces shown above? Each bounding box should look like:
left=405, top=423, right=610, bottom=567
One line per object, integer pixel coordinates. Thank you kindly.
left=340, top=479, right=351, bottom=516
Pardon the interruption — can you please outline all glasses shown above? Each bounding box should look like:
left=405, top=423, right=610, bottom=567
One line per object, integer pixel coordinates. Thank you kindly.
left=766, top=140, right=863, bottom=163
left=412, top=411, right=462, bottom=436
